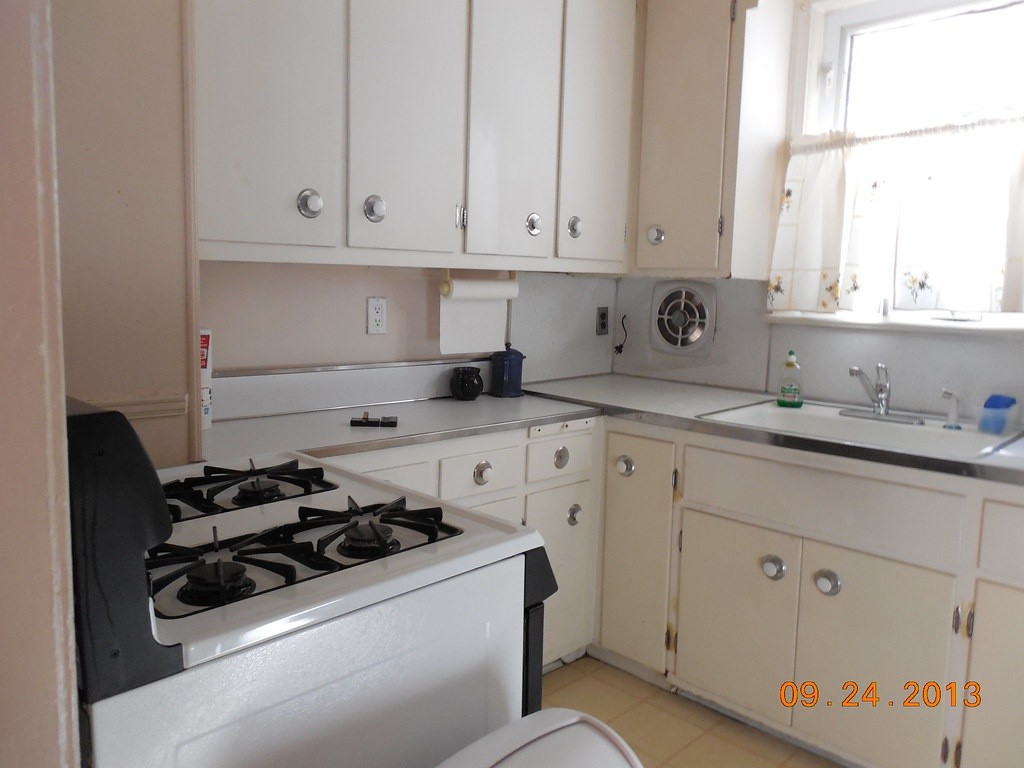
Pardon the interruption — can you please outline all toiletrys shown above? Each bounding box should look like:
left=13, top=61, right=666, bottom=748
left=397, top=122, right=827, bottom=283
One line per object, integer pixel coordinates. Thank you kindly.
left=776, top=350, right=803, bottom=407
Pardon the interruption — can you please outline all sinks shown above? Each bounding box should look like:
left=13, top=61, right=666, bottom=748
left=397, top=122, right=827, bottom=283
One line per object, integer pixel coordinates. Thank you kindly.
left=712, top=404, right=995, bottom=462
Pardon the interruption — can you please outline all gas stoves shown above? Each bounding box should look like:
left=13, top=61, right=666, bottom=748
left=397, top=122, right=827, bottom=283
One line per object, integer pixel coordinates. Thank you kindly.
left=142, top=453, right=468, bottom=625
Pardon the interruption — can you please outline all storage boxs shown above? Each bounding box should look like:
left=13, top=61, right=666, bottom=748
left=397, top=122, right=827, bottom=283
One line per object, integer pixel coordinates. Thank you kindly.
left=489, top=342, right=526, bottom=399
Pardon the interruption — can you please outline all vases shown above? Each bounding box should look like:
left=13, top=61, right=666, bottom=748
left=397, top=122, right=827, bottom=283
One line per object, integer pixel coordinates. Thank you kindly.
left=449, top=367, right=484, bottom=401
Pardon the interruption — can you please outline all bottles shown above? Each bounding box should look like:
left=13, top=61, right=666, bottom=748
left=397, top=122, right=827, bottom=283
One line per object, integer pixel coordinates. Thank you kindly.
left=778, top=351, right=804, bottom=406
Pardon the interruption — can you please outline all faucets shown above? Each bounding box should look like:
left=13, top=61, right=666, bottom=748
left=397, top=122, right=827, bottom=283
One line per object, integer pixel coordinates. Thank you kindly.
left=849, top=365, right=890, bottom=414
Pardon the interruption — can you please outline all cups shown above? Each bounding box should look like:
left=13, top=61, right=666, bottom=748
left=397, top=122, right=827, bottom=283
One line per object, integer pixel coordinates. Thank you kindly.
left=489, top=344, right=526, bottom=396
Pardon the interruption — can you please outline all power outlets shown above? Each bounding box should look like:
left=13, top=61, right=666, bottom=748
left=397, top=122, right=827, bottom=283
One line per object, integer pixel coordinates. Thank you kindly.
left=596, top=307, right=609, bottom=335
left=367, top=296, right=388, bottom=335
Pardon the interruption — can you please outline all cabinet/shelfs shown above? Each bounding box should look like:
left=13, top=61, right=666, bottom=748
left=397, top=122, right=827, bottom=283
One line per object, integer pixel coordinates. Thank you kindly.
left=194, top=0, right=797, bottom=283
left=310, top=412, right=1024, bottom=768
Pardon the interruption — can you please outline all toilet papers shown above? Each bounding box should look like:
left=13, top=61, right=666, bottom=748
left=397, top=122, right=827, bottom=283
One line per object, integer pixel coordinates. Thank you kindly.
left=439, top=279, right=519, bottom=355
